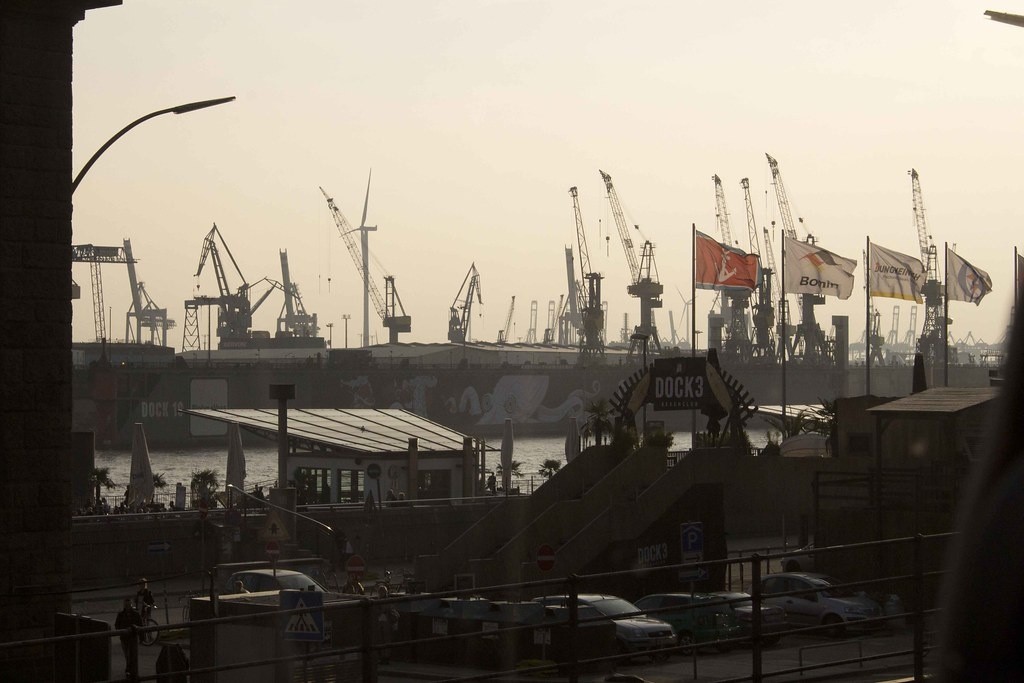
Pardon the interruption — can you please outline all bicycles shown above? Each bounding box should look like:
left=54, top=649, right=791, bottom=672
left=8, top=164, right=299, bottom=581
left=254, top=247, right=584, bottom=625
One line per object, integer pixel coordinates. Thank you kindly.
left=179, top=587, right=200, bottom=623
left=131, top=599, right=160, bottom=647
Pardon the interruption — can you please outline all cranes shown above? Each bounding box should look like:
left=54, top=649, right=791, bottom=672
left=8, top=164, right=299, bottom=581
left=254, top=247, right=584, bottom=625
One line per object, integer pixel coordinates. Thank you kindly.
left=70, top=150, right=953, bottom=367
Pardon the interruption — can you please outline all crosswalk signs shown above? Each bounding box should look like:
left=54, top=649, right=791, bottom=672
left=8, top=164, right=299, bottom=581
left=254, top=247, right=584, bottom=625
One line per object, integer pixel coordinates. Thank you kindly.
left=276, top=590, right=325, bottom=644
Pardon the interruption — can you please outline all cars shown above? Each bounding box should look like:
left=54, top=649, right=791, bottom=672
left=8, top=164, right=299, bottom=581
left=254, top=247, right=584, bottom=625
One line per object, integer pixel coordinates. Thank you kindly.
left=530, top=593, right=676, bottom=667
left=224, top=569, right=329, bottom=594
left=705, top=591, right=790, bottom=648
left=746, top=571, right=886, bottom=639
left=633, top=593, right=741, bottom=658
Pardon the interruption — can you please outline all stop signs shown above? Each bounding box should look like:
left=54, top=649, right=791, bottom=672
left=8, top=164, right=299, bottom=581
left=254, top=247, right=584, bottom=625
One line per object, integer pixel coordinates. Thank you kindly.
left=345, top=554, right=366, bottom=580
left=536, top=543, right=558, bottom=575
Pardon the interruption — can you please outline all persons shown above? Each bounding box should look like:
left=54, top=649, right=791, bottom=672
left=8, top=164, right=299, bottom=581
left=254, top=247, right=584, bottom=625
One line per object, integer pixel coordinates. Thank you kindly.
left=135, top=578, right=154, bottom=643
left=115, top=599, right=143, bottom=678
left=386, top=489, right=409, bottom=507
left=934, top=298, right=1024, bottom=683
left=248, top=485, right=267, bottom=507
left=72, top=498, right=176, bottom=522
left=121, top=485, right=130, bottom=507
left=234, top=581, right=250, bottom=594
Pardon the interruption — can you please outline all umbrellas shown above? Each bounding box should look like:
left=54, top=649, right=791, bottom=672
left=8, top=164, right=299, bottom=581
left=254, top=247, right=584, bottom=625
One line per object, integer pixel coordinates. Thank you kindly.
left=129, top=424, right=154, bottom=499
left=565, top=417, right=581, bottom=462
left=501, top=421, right=512, bottom=490
left=226, top=421, right=247, bottom=505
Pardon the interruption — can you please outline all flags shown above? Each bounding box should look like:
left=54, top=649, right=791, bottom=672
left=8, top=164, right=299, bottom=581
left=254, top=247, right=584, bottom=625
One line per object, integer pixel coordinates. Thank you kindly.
left=1017, top=254, right=1024, bottom=302
left=785, top=236, right=857, bottom=300
left=947, top=248, right=993, bottom=306
left=696, top=230, right=764, bottom=292
left=870, top=242, right=927, bottom=304
